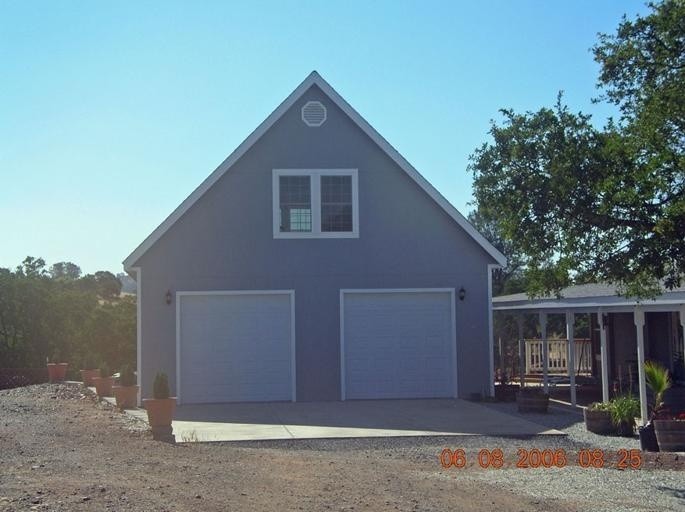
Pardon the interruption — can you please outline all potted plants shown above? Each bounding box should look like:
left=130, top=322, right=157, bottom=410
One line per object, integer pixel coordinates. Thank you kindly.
left=584, top=399, right=612, bottom=434
left=143, top=370, right=179, bottom=431
left=44, top=350, right=141, bottom=410
left=636, top=356, right=672, bottom=450
left=609, top=391, right=637, bottom=437
left=494, top=366, right=549, bottom=414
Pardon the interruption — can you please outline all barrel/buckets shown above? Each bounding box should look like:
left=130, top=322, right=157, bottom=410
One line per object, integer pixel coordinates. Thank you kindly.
left=47, top=363, right=69, bottom=383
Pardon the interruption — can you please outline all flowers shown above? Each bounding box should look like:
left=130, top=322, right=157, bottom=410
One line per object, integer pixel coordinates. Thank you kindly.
left=649, top=402, right=685, bottom=422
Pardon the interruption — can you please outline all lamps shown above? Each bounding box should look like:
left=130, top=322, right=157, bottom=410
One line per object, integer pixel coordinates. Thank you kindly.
left=164, top=287, right=172, bottom=305
left=458, top=284, right=468, bottom=302
